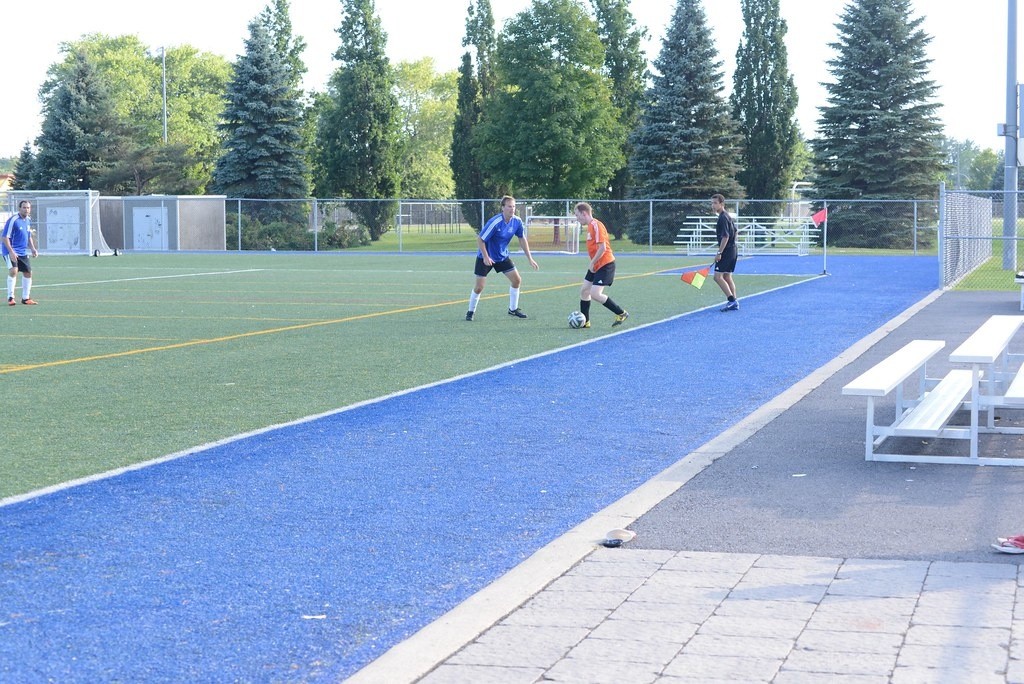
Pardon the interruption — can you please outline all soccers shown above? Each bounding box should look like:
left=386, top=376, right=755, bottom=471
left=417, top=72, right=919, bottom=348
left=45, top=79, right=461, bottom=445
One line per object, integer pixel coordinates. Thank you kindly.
left=567, top=311, right=586, bottom=328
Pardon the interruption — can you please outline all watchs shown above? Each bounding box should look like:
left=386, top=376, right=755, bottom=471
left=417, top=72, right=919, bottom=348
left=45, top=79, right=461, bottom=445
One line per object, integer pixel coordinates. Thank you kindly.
left=717, top=252, right=722, bottom=255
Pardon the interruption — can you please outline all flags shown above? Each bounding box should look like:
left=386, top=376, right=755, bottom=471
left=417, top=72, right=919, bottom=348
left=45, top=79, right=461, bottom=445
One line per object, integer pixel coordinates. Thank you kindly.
left=811, top=208, right=827, bottom=229
left=681, top=267, right=711, bottom=290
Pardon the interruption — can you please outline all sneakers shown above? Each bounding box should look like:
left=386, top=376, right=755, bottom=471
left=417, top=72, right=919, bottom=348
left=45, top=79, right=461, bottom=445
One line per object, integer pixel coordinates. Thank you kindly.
left=466, top=311, right=475, bottom=321
left=611, top=310, right=630, bottom=327
left=21, top=298, right=39, bottom=305
left=7, top=297, right=15, bottom=305
left=569, top=320, right=590, bottom=328
left=719, top=299, right=739, bottom=311
left=507, top=307, right=528, bottom=319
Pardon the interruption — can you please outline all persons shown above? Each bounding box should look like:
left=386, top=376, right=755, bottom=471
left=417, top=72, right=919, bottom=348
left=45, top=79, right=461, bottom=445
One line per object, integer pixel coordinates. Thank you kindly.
left=711, top=194, right=740, bottom=312
left=465, top=195, right=539, bottom=321
left=1, top=200, right=39, bottom=306
left=574, top=201, right=630, bottom=328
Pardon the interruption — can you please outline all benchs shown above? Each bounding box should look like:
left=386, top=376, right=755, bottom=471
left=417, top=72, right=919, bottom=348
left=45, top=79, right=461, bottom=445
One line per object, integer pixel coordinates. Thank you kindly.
left=842, top=314, right=1024, bottom=466
left=673, top=216, right=821, bottom=257
